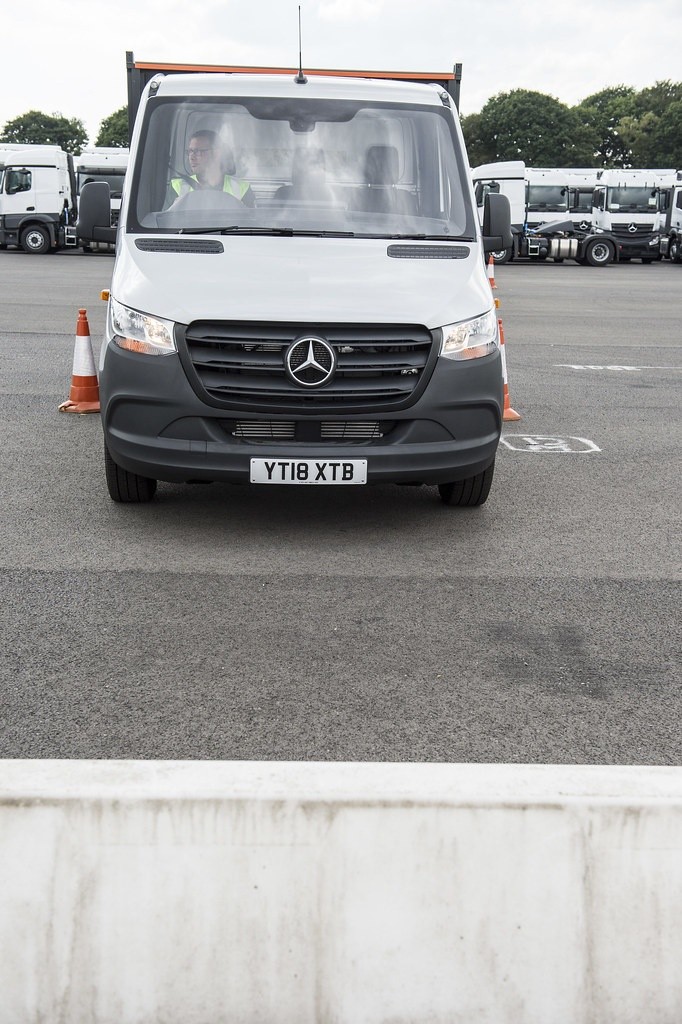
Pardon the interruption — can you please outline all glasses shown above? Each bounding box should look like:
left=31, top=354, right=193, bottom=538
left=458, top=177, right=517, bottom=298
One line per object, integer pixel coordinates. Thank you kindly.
left=187, top=149, right=213, bottom=156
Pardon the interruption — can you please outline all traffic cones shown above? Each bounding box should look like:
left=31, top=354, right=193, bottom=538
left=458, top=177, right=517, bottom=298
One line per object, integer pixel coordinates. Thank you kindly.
left=485, top=257, right=496, bottom=287
left=496, top=318, right=520, bottom=423
left=58, top=307, right=102, bottom=415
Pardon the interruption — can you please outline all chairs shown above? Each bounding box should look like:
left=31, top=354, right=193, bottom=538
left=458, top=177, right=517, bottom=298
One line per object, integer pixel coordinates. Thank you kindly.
left=274, top=145, right=336, bottom=208
left=350, top=146, right=417, bottom=215
left=223, top=144, right=238, bottom=177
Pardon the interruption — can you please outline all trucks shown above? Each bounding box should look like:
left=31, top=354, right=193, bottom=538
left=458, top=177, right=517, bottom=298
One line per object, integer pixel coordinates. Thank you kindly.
left=470, top=160, right=620, bottom=267
left=0, top=141, right=131, bottom=254
left=526, top=166, right=682, bottom=263
left=97, top=50, right=504, bottom=505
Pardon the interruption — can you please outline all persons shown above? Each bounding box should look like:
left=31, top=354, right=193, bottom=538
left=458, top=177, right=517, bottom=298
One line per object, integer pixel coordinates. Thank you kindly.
left=162, top=130, right=257, bottom=211
